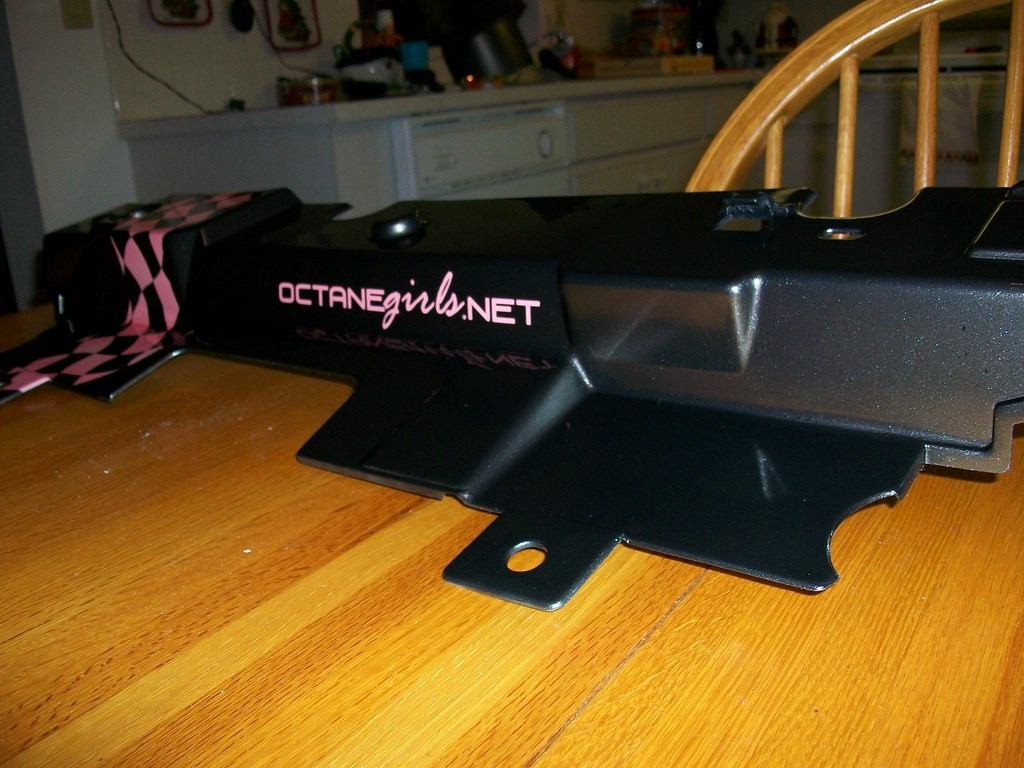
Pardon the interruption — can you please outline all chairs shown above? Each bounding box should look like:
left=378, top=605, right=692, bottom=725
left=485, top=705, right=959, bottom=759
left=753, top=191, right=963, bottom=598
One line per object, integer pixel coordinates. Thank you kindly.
left=680, top=0, right=1024, bottom=217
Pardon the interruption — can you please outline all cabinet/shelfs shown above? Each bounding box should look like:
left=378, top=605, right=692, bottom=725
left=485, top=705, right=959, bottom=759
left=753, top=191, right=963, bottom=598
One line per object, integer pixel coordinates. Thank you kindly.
left=119, top=69, right=1023, bottom=222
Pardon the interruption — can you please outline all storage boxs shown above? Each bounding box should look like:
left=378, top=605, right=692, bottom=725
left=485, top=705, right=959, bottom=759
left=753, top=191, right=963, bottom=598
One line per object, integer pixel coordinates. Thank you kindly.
left=586, top=55, right=714, bottom=75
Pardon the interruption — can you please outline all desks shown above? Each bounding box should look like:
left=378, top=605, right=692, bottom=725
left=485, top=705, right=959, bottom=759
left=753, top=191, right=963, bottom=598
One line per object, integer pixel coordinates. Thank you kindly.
left=1, top=301, right=1023, bottom=766
